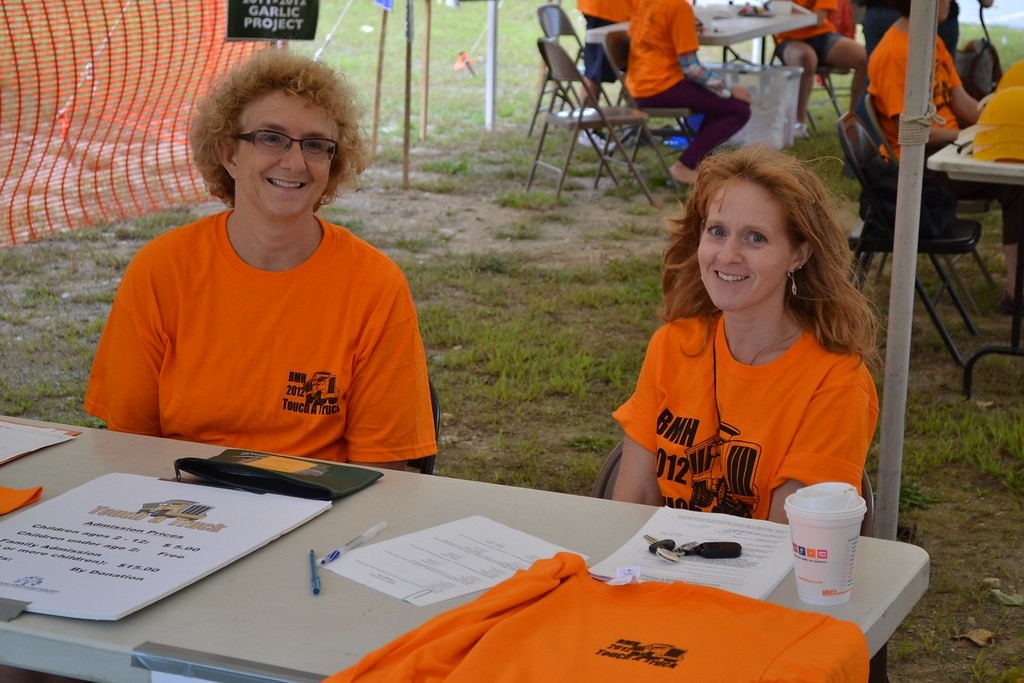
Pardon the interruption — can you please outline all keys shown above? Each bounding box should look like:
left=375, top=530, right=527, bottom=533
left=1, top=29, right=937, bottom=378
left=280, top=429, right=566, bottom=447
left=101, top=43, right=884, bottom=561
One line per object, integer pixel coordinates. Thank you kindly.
left=645, top=535, right=676, bottom=554
left=697, top=542, right=743, bottom=559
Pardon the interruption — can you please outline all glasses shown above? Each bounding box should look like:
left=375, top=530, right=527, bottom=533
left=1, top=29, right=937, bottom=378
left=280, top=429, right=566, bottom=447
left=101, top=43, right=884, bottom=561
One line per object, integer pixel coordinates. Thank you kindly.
left=229, top=129, right=338, bottom=161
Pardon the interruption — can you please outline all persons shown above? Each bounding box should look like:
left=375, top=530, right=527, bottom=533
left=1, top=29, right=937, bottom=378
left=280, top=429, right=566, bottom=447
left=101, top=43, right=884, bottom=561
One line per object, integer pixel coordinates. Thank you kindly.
left=612, top=143, right=879, bottom=524
left=576, top=0, right=1024, bottom=314
left=81, top=49, right=438, bottom=471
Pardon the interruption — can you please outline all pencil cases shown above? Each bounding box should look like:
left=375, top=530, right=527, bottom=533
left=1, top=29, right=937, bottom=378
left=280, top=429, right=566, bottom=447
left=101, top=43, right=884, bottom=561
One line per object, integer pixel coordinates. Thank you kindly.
left=174, top=449, right=384, bottom=501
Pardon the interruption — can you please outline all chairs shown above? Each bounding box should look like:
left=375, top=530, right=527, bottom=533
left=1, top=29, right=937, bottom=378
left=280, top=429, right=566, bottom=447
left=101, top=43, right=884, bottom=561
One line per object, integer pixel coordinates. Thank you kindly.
left=763, top=0, right=850, bottom=134
left=525, top=36, right=656, bottom=209
left=837, top=110, right=977, bottom=370
left=866, top=92, right=996, bottom=317
left=526, top=4, right=625, bottom=136
left=594, top=28, right=697, bottom=185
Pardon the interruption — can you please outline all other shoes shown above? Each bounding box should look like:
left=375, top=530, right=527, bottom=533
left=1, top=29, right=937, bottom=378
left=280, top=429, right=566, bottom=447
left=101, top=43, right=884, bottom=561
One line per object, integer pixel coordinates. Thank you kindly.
left=792, top=123, right=810, bottom=141
left=997, top=292, right=1015, bottom=315
left=579, top=130, right=617, bottom=151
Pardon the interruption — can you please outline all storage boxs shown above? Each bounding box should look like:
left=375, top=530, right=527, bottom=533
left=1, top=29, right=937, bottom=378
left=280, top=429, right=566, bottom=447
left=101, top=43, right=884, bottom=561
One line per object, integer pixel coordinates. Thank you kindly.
left=703, top=63, right=804, bottom=151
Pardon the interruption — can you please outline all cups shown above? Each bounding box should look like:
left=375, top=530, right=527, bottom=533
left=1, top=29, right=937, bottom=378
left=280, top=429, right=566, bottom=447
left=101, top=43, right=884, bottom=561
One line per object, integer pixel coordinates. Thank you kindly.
left=783, top=481, right=867, bottom=606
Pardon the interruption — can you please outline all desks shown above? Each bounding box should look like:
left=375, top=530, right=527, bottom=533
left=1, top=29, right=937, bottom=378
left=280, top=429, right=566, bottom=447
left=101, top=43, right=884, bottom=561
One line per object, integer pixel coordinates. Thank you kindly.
left=0, top=415, right=932, bottom=683
left=694, top=5, right=818, bottom=66
left=926, top=123, right=1024, bottom=400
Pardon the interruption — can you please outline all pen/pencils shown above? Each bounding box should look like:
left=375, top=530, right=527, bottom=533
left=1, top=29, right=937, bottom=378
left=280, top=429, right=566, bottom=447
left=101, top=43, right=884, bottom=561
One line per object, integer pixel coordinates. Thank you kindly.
left=309, top=550, right=321, bottom=594
left=321, top=522, right=387, bottom=566
